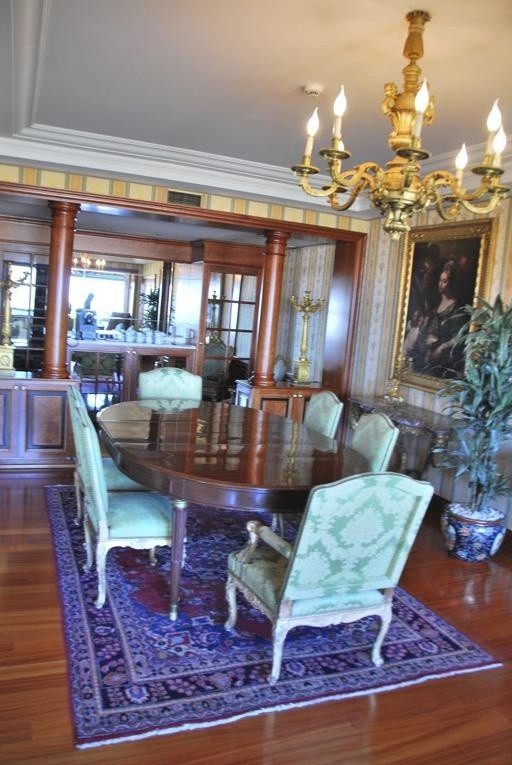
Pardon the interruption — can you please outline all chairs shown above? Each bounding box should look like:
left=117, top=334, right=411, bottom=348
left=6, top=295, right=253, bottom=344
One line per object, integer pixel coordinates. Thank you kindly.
left=66, top=384, right=158, bottom=525
left=270, top=390, right=345, bottom=531
left=351, top=409, right=400, bottom=472
left=220, top=354, right=289, bottom=405
left=223, top=473, right=435, bottom=686
left=138, top=366, right=203, bottom=400
left=70, top=405, right=188, bottom=609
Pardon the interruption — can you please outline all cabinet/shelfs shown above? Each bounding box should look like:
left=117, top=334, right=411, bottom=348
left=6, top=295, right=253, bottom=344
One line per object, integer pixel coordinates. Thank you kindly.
left=67, top=340, right=198, bottom=426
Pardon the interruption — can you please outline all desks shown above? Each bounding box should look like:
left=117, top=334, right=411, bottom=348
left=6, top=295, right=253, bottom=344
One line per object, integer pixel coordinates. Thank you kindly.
left=345, top=396, right=468, bottom=480
left=95, top=397, right=373, bottom=620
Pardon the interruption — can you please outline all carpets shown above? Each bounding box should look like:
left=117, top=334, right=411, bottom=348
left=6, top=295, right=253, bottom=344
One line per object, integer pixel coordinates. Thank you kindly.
left=46, top=483, right=504, bottom=750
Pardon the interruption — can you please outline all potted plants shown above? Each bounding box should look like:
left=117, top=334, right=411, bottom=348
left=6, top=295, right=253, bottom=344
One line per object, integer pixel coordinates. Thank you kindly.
left=427, top=294, right=511, bottom=562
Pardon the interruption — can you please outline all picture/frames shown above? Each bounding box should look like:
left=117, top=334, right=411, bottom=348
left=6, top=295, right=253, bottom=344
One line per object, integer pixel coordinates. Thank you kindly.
left=388, top=217, right=499, bottom=396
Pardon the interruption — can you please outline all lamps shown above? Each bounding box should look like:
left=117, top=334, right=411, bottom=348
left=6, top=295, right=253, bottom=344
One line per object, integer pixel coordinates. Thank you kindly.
left=292, top=10, right=511, bottom=241
left=71, top=252, right=106, bottom=270
